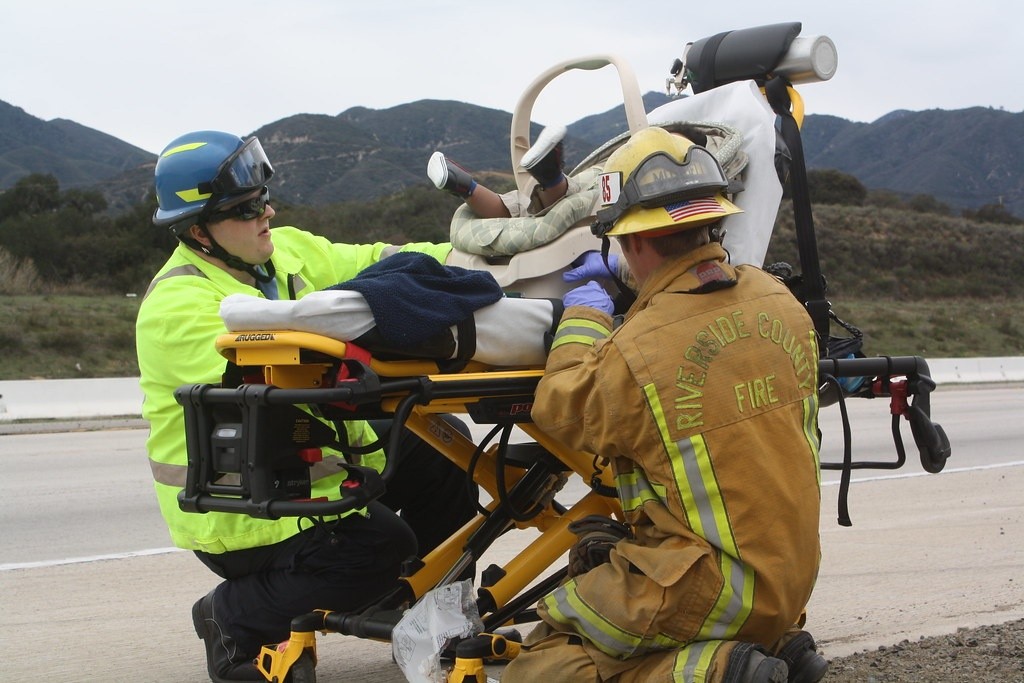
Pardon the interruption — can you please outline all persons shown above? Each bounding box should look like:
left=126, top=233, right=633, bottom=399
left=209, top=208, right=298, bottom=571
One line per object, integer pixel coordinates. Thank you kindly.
left=500, top=125, right=831, bottom=683
left=427, top=123, right=707, bottom=219
left=134, top=132, right=454, bottom=682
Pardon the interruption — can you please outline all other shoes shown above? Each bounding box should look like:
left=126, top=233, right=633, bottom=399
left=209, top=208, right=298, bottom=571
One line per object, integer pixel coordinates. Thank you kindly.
left=426, top=151, right=472, bottom=203
left=518, top=114, right=570, bottom=188
left=719, top=632, right=828, bottom=683
left=192, top=587, right=266, bottom=683
left=392, top=628, right=522, bottom=664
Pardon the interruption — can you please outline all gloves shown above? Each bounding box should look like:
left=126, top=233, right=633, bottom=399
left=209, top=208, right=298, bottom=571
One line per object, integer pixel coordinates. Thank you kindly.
left=563, top=252, right=619, bottom=282
left=560, top=277, right=616, bottom=317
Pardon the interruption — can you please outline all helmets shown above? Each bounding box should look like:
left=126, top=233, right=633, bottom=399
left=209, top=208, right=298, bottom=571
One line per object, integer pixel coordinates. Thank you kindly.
left=155, top=132, right=273, bottom=221
left=593, top=126, right=747, bottom=239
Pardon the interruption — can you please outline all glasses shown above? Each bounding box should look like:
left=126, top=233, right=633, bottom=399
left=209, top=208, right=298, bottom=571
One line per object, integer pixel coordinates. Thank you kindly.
left=616, top=144, right=731, bottom=211
left=208, top=184, right=270, bottom=222
